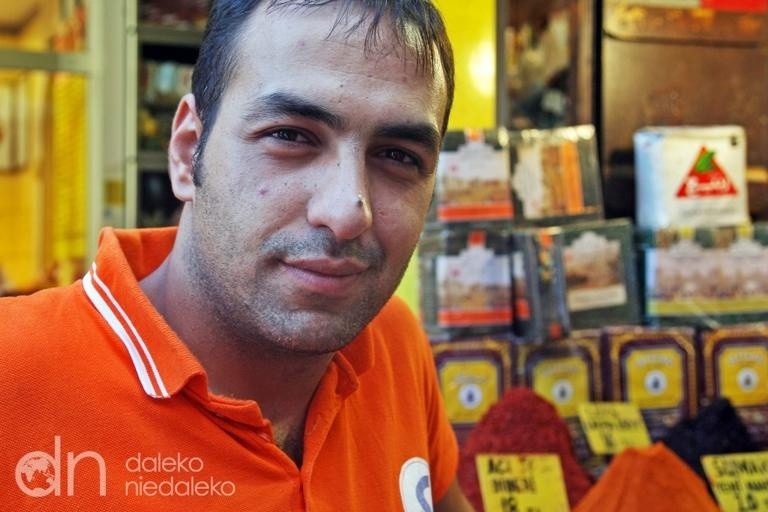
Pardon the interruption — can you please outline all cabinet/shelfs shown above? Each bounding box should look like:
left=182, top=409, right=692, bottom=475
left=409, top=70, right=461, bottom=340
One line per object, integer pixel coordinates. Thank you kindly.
left=139, top=23, right=207, bottom=228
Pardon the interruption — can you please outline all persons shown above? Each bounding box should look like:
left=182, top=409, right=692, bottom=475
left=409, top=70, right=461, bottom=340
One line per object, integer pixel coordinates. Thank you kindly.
left=0, top=1, right=476, bottom=512
